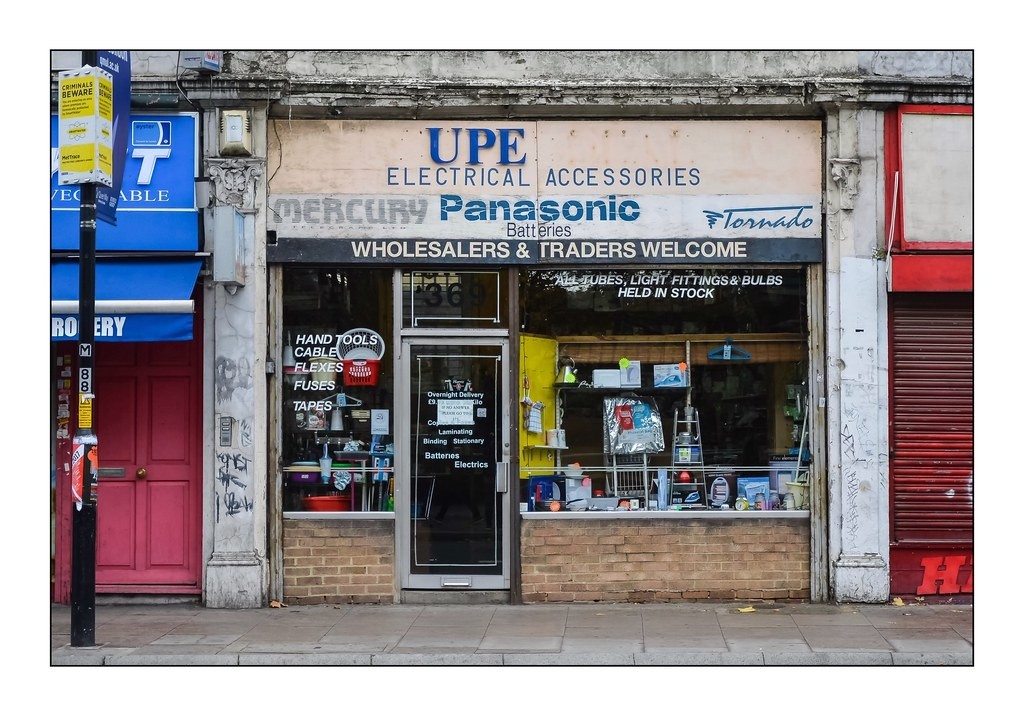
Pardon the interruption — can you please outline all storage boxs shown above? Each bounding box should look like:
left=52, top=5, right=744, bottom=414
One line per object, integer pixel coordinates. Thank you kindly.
left=591, top=369, right=621, bottom=388
left=737, top=477, right=770, bottom=510
left=653, top=365, right=687, bottom=387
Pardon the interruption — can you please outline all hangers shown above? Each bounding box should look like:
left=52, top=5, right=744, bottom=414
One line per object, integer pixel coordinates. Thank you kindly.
left=707, top=336, right=752, bottom=360
left=320, top=385, right=363, bottom=407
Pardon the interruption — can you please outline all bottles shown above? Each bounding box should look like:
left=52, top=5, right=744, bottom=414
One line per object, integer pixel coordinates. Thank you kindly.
left=768, top=490, right=780, bottom=510
left=755, top=493, right=766, bottom=511
left=783, top=490, right=795, bottom=511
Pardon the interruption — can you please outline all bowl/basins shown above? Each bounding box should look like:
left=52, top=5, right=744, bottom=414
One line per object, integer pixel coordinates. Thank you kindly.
left=301, top=497, right=352, bottom=512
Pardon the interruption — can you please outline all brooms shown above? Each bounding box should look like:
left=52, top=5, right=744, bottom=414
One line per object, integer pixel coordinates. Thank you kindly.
left=333, top=450, right=369, bottom=512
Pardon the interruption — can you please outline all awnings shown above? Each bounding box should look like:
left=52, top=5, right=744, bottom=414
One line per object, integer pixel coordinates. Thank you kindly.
left=52, top=259, right=203, bottom=342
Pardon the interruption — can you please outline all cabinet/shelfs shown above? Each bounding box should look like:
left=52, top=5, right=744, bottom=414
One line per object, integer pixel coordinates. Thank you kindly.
left=720, top=393, right=769, bottom=430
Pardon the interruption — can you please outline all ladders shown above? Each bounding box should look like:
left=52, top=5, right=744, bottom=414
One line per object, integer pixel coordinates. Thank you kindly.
left=669, top=407, right=710, bottom=509
